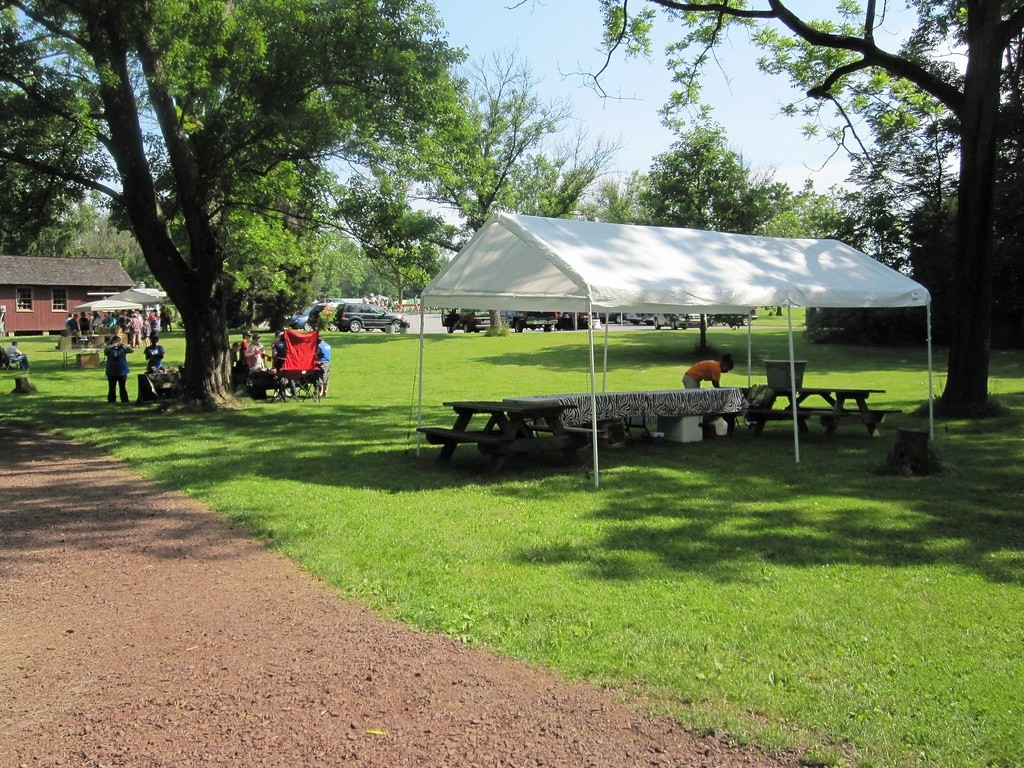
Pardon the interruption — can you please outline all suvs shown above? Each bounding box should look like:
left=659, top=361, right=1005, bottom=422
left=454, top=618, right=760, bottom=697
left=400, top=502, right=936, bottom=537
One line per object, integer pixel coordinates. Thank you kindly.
left=333, top=303, right=410, bottom=333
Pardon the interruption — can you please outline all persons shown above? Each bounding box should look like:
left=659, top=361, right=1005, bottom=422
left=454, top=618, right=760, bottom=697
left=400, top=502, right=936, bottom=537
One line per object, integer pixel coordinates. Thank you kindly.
left=6, top=340, right=29, bottom=369
left=90, top=312, right=100, bottom=330
left=246, top=332, right=265, bottom=371
left=78, top=312, right=89, bottom=334
left=313, top=337, right=331, bottom=397
left=65, top=312, right=73, bottom=329
left=66, top=314, right=79, bottom=342
left=230, top=341, right=240, bottom=394
left=104, top=336, right=134, bottom=404
left=126, top=314, right=142, bottom=348
left=0, top=305, right=5, bottom=338
left=143, top=331, right=165, bottom=374
left=273, top=333, right=297, bottom=403
left=102, top=312, right=115, bottom=328
left=682, top=353, right=734, bottom=389
left=271, top=331, right=283, bottom=346
left=114, top=305, right=172, bottom=347
left=113, top=310, right=119, bottom=318
left=241, top=331, right=258, bottom=365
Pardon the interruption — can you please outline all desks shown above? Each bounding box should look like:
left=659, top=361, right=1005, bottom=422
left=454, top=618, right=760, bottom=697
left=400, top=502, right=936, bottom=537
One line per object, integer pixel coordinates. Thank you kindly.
left=435, top=399, right=582, bottom=476
left=57, top=347, right=106, bottom=370
left=59, top=334, right=128, bottom=350
left=721, top=386, right=886, bottom=436
left=504, top=385, right=768, bottom=438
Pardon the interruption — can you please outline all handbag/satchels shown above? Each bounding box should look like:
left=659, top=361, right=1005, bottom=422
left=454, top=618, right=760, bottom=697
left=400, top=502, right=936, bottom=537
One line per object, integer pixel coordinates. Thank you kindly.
left=123, top=327, right=130, bottom=333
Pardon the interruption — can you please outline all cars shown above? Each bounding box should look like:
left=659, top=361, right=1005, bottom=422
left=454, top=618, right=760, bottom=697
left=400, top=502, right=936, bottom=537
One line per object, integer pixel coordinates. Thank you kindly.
left=291, top=298, right=368, bottom=332
left=707, top=310, right=757, bottom=327
left=596, top=313, right=707, bottom=329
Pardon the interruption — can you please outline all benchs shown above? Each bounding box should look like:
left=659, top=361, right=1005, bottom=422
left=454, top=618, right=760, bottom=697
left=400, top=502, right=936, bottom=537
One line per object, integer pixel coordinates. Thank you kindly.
left=416, top=424, right=605, bottom=448
left=747, top=407, right=902, bottom=421
left=526, top=424, right=605, bottom=447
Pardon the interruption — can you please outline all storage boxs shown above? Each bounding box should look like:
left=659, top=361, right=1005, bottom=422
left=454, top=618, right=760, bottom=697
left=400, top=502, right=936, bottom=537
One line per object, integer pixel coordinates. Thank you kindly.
left=764, top=360, right=809, bottom=388
left=75, top=353, right=99, bottom=368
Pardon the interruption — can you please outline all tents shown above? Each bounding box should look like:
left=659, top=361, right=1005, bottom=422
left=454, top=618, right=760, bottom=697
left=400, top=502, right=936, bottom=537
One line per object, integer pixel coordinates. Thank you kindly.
left=72, top=288, right=167, bottom=333
left=421, top=212, right=934, bottom=489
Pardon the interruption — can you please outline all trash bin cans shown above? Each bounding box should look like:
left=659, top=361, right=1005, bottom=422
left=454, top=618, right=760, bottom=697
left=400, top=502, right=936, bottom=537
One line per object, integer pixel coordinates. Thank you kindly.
left=384, top=323, right=395, bottom=333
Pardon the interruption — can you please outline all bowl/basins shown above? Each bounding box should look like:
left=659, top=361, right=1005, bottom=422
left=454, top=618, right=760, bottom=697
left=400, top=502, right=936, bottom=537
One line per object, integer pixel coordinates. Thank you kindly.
left=764, top=361, right=808, bottom=389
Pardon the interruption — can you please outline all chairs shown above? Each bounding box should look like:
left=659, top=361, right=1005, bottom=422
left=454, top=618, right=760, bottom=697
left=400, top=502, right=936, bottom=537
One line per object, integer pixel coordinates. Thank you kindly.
left=270, top=328, right=323, bottom=403
left=623, top=416, right=652, bottom=443
left=0, top=346, right=28, bottom=369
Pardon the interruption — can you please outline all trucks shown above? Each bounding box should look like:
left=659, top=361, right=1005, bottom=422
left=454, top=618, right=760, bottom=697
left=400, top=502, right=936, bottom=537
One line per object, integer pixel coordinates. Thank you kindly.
left=442, top=310, right=592, bottom=335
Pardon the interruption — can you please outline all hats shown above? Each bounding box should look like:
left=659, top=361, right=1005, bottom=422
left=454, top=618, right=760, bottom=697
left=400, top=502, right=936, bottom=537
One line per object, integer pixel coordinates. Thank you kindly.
left=243, top=330, right=252, bottom=338
left=148, top=331, right=159, bottom=338
left=252, top=333, right=261, bottom=339
